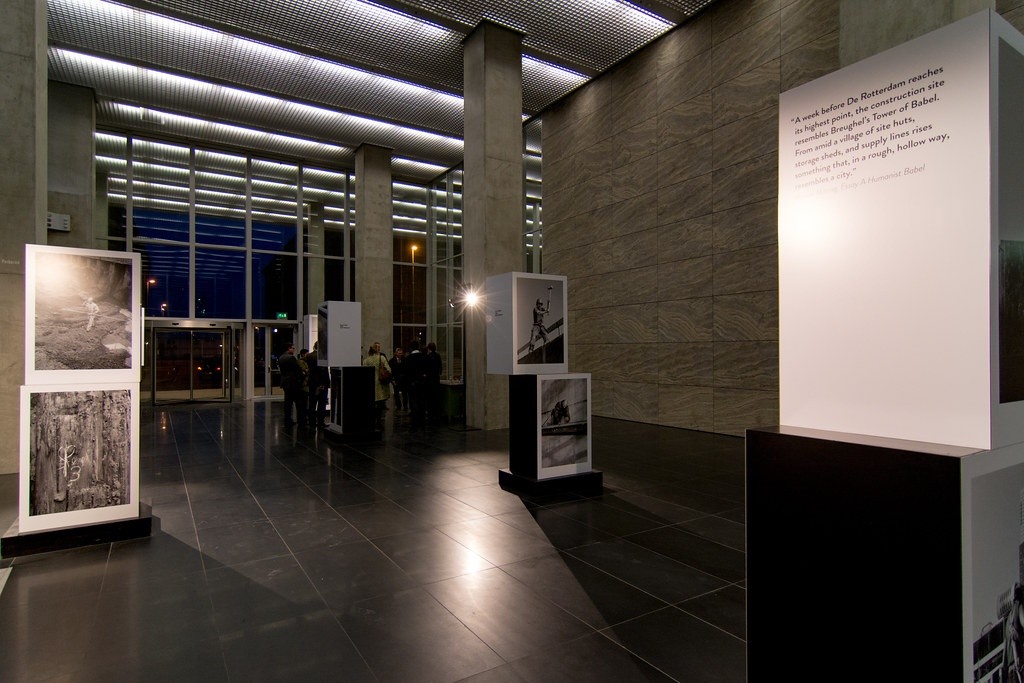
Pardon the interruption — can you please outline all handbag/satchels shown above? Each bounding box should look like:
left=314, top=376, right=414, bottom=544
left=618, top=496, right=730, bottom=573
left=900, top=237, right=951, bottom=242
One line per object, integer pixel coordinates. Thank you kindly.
left=379, top=355, right=393, bottom=383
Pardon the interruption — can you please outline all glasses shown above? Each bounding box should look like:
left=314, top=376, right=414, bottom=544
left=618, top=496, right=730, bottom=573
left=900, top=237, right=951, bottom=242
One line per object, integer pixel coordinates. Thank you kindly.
left=291, top=348, right=295, bottom=349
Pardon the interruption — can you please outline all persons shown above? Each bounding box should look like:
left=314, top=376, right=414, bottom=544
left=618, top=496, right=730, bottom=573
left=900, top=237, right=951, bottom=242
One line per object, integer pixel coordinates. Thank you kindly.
left=529, top=285, right=553, bottom=351
left=278, top=339, right=443, bottom=433
left=453, top=375, right=461, bottom=384
left=551, top=399, right=571, bottom=426
left=82, top=297, right=100, bottom=332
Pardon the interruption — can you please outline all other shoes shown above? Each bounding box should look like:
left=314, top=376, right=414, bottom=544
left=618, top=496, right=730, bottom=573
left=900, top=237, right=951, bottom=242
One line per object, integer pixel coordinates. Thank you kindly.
left=383, top=405, right=408, bottom=411
left=284, top=422, right=296, bottom=426
left=299, top=421, right=330, bottom=428
